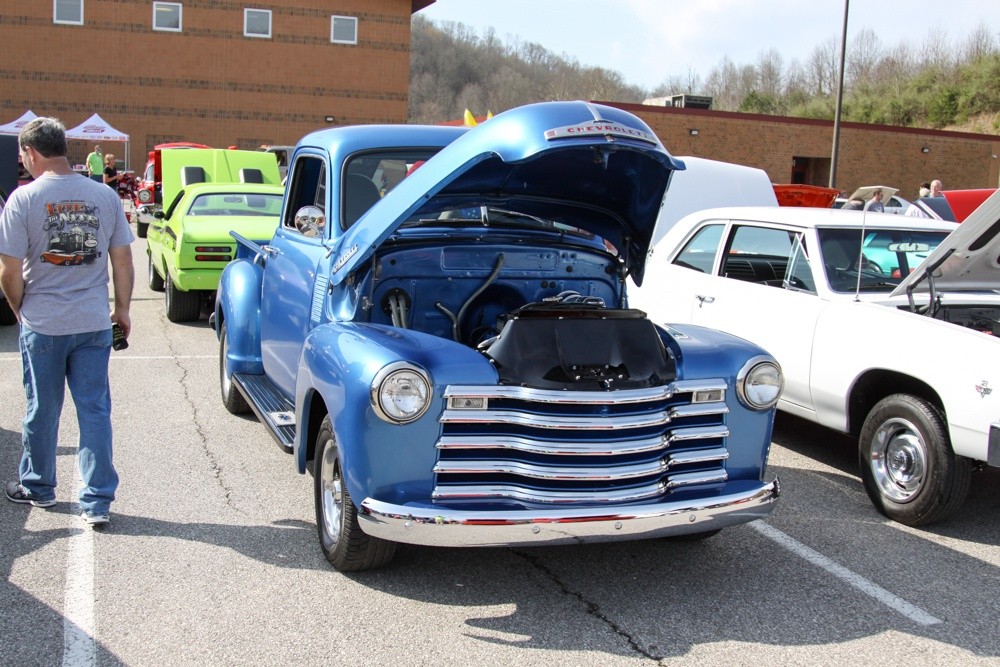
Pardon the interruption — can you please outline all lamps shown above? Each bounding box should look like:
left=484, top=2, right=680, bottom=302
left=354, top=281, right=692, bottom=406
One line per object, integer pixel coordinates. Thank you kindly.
left=923, top=147, right=929, bottom=152
left=691, top=130, right=699, bottom=135
left=326, top=115, right=334, bottom=122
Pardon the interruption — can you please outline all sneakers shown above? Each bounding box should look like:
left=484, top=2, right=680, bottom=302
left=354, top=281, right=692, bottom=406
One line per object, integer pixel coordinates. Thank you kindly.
left=81, top=509, right=110, bottom=523
left=5, top=480, right=57, bottom=506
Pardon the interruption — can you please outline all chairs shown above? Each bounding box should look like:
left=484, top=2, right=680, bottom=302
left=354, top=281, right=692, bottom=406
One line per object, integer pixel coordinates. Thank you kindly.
left=344, top=172, right=382, bottom=230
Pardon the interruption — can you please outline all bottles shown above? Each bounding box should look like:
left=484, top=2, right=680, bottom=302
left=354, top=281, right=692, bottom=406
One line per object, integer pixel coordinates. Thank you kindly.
left=112, top=322, right=128, bottom=351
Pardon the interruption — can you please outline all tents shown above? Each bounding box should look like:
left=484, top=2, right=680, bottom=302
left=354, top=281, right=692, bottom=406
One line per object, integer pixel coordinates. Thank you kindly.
left=0, top=109, right=130, bottom=172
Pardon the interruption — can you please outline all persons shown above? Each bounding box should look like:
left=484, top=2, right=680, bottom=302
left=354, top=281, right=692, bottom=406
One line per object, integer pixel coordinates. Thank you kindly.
left=862, top=180, right=944, bottom=218
left=85, top=145, right=122, bottom=194
left=0, top=116, right=135, bottom=527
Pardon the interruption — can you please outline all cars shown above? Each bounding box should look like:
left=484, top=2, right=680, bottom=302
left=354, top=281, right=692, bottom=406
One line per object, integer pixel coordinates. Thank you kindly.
left=134, top=142, right=213, bottom=238
left=210, top=99, right=785, bottom=573
left=773, top=183, right=997, bottom=276
left=620, top=155, right=999, bottom=529
left=254, top=146, right=297, bottom=181
left=145, top=148, right=288, bottom=324
left=0, top=134, right=35, bottom=325
